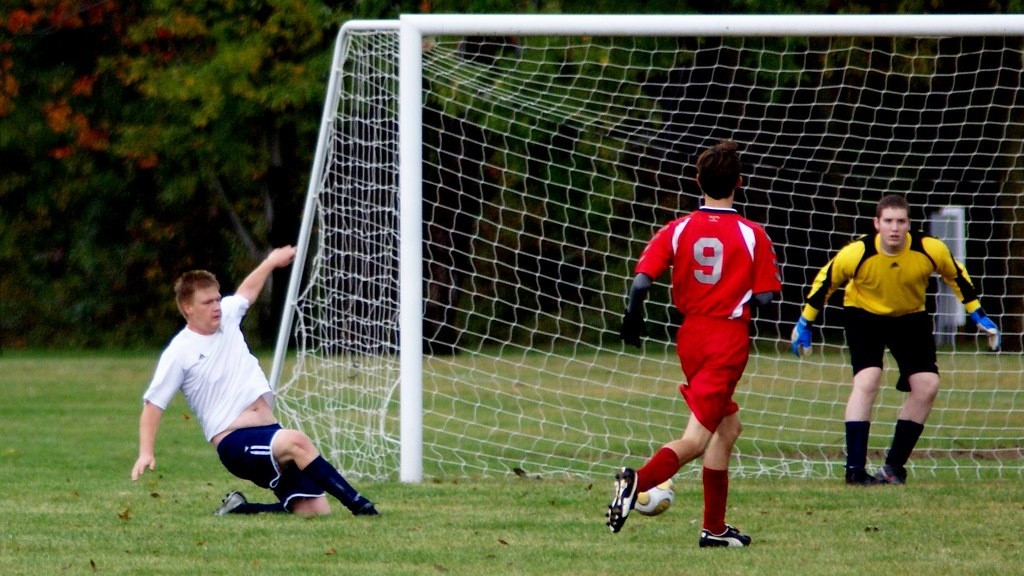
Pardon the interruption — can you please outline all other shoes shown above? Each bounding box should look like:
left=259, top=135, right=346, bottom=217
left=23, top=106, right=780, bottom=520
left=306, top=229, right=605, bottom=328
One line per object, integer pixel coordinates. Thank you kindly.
left=844, top=470, right=890, bottom=486
left=875, top=465, right=903, bottom=485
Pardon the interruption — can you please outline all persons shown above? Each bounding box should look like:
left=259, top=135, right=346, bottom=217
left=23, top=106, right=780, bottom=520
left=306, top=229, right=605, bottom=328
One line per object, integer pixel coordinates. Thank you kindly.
left=605, top=138, right=783, bottom=547
left=132, top=244, right=379, bottom=516
left=792, top=195, right=1002, bottom=487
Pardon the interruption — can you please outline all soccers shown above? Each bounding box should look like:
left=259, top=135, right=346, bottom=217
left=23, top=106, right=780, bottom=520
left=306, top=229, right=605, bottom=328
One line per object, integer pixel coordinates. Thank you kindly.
left=633, top=477, right=675, bottom=516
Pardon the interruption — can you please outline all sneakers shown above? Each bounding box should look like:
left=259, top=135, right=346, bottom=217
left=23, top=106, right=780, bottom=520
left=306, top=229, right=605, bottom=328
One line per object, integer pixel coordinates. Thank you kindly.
left=699, top=524, right=751, bottom=548
left=605, top=466, right=638, bottom=533
left=212, top=489, right=247, bottom=516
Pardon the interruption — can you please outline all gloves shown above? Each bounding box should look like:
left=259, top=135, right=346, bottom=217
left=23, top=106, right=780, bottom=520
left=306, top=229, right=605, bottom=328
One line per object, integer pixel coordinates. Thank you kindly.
left=969, top=306, right=1001, bottom=350
left=619, top=309, right=649, bottom=348
left=791, top=315, right=814, bottom=358
